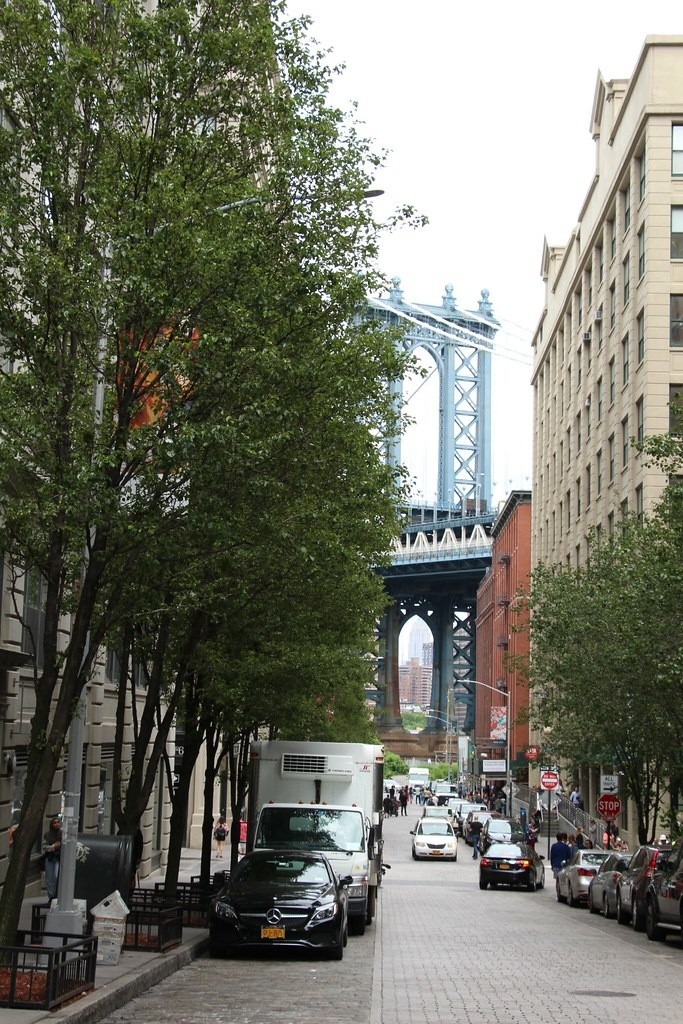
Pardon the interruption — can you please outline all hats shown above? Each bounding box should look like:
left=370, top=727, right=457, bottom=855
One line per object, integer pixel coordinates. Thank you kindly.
left=660, top=835, right=666, bottom=841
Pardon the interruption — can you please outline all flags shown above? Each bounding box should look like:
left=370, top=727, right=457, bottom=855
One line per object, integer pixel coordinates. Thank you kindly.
left=117, top=308, right=199, bottom=432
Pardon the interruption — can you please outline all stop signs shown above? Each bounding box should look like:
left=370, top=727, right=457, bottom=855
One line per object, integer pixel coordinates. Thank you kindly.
left=540, top=770, right=559, bottom=791
left=597, top=792, right=622, bottom=819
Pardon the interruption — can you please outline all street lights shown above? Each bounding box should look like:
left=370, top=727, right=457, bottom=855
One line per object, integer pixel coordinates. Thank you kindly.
left=456, top=679, right=510, bottom=818
left=38, top=188, right=385, bottom=984
left=424, top=715, right=453, bottom=784
left=428, top=708, right=459, bottom=795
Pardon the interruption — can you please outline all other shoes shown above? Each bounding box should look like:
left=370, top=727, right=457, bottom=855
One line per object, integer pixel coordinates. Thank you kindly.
left=48, top=898, right=52, bottom=905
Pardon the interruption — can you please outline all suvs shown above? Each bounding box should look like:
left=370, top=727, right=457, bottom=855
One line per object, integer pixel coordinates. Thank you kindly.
left=646, top=836, right=682, bottom=948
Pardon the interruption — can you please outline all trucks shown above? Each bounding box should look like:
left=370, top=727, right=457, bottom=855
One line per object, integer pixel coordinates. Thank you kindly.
left=407, top=767, right=430, bottom=790
left=247, top=739, right=393, bottom=937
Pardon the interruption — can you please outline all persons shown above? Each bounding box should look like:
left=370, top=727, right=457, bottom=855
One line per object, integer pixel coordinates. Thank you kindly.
left=415, top=785, right=443, bottom=806
left=570, top=787, right=581, bottom=815
left=213, top=816, right=229, bottom=859
left=652, top=834, right=676, bottom=845
left=457, top=780, right=506, bottom=815
left=514, top=808, right=542, bottom=851
left=383, top=785, right=414, bottom=818
left=116, top=808, right=144, bottom=910
left=237, top=813, right=248, bottom=857
left=42, top=817, right=62, bottom=905
left=550, top=819, right=628, bottom=902
left=469, top=816, right=483, bottom=860
left=554, top=778, right=566, bottom=797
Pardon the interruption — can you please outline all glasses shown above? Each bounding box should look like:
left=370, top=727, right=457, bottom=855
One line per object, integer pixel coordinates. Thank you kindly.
left=585, top=842, right=588, bottom=845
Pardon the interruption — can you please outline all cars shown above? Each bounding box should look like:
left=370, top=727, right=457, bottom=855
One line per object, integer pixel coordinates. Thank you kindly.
left=424, top=780, right=489, bottom=837
left=479, top=843, right=545, bottom=892
left=409, top=816, right=461, bottom=862
left=613, top=844, right=675, bottom=933
left=587, top=852, right=634, bottom=921
left=382, top=778, right=402, bottom=794
left=209, top=848, right=354, bottom=961
left=555, top=848, right=613, bottom=909
left=479, top=817, right=531, bottom=856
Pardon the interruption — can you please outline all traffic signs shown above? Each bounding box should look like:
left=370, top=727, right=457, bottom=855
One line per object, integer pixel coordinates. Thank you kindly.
left=600, top=775, right=618, bottom=795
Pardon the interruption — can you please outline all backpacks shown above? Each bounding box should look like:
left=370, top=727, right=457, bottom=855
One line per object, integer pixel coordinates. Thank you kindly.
left=215, top=825, right=225, bottom=841
